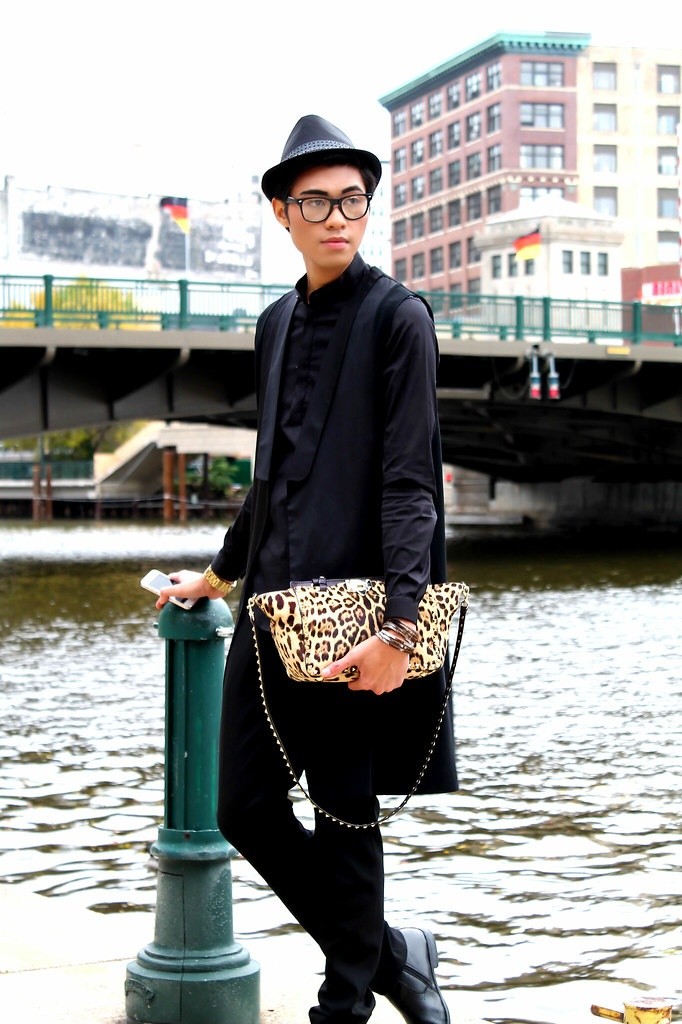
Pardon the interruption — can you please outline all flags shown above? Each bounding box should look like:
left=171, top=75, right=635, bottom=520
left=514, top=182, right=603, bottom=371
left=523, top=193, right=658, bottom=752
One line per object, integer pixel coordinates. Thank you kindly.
left=511, top=229, right=541, bottom=261
left=162, top=197, right=189, bottom=232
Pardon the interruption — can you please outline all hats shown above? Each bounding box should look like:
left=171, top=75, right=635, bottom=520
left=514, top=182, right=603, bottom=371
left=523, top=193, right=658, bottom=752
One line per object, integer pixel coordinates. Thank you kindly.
left=260, top=113, right=383, bottom=204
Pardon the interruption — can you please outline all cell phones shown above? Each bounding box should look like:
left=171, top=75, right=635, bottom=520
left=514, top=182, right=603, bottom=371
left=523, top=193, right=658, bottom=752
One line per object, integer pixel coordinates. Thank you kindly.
left=141, top=570, right=199, bottom=610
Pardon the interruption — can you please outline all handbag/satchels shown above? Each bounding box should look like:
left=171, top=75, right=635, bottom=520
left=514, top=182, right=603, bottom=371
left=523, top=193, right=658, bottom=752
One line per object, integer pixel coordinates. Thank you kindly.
left=254, top=576, right=470, bottom=684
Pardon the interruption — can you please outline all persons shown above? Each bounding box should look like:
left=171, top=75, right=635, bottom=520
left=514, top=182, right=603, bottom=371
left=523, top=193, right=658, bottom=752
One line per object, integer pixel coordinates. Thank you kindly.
left=154, top=114, right=460, bottom=1024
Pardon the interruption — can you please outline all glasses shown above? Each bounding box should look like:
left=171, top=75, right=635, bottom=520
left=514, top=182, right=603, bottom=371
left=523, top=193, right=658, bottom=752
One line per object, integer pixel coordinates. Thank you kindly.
left=285, top=191, right=373, bottom=223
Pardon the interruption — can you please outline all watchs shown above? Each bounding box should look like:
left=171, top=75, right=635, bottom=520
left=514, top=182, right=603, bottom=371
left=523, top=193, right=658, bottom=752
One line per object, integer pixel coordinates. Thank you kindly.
left=204, top=564, right=238, bottom=595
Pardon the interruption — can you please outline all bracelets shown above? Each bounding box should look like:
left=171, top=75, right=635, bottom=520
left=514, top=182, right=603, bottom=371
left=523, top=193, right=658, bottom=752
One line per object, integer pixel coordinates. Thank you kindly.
left=373, top=628, right=414, bottom=654
left=382, top=619, right=422, bottom=643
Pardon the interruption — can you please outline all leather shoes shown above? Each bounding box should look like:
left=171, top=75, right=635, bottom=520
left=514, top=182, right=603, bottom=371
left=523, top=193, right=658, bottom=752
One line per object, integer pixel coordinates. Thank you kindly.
left=384, top=925, right=451, bottom=1024
